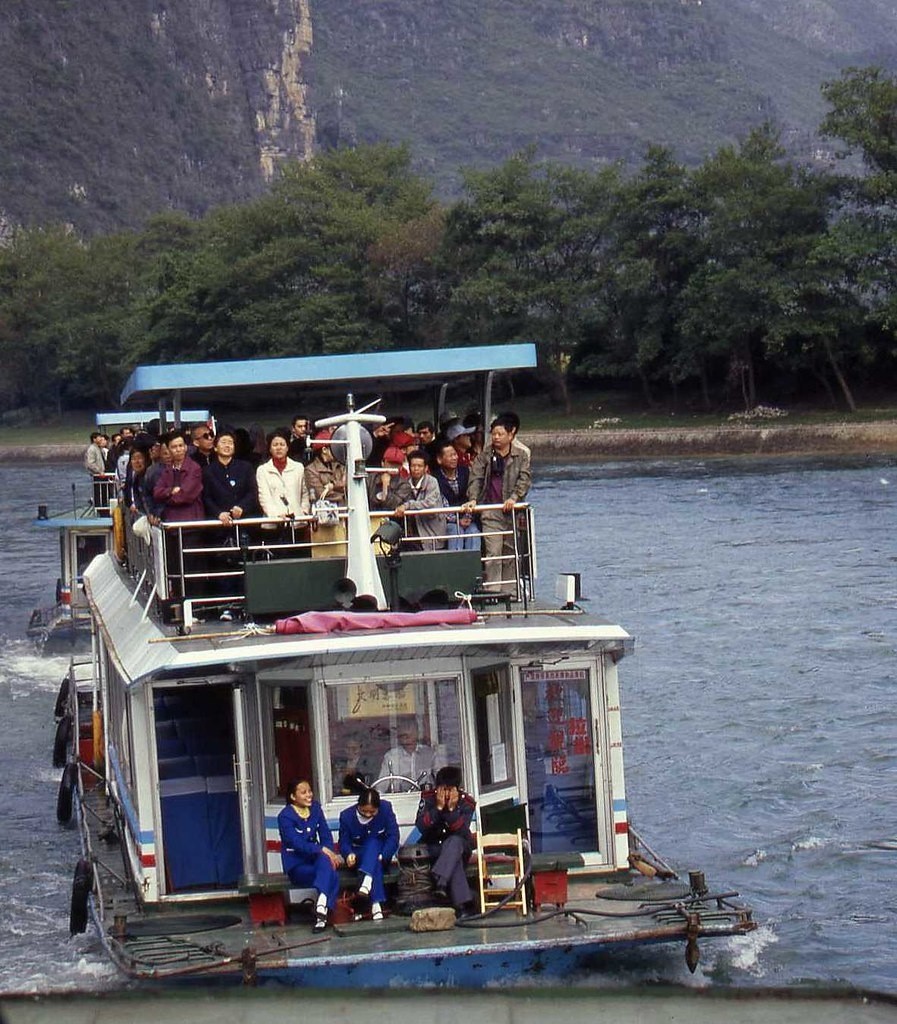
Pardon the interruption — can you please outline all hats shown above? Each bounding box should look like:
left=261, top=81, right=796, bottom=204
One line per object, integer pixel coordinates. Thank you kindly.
left=312, top=432, right=331, bottom=449
left=446, top=425, right=476, bottom=440
left=132, top=516, right=151, bottom=547
left=392, top=431, right=426, bottom=449
left=463, top=411, right=485, bottom=428
left=383, top=447, right=404, bottom=464
left=439, top=411, right=461, bottom=428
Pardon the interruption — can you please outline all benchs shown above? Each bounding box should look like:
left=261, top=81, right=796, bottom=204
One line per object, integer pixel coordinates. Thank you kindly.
left=237, top=850, right=585, bottom=929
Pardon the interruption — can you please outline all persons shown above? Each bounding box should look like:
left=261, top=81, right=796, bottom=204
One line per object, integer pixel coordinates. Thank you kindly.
left=305, top=428, right=347, bottom=559
left=278, top=776, right=344, bottom=932
left=374, top=714, right=441, bottom=791
left=415, top=767, right=477, bottom=919
left=203, top=433, right=259, bottom=620
left=366, top=446, right=422, bottom=551
left=392, top=450, right=448, bottom=552
left=154, top=432, right=207, bottom=623
left=84, top=409, right=532, bottom=625
left=338, top=789, right=400, bottom=920
left=190, top=425, right=217, bottom=488
left=256, top=431, right=310, bottom=556
left=330, top=731, right=384, bottom=794
left=461, top=417, right=531, bottom=595
left=84, top=433, right=109, bottom=516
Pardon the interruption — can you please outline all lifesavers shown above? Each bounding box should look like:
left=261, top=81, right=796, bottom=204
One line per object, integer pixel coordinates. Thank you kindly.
left=58, top=764, right=78, bottom=823
left=56, top=679, right=69, bottom=716
left=53, top=715, right=71, bottom=768
left=70, top=862, right=95, bottom=934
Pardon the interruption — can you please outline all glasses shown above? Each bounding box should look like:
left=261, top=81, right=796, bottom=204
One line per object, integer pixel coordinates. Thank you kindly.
left=196, top=430, right=214, bottom=440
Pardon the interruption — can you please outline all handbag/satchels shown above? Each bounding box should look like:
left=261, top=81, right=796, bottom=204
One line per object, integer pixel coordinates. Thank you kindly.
left=316, top=488, right=339, bottom=527
left=213, top=538, right=246, bottom=567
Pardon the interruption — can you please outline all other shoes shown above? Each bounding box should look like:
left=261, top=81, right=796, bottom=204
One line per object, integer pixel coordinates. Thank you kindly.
left=356, top=886, right=371, bottom=897
left=431, top=885, right=447, bottom=898
left=313, top=921, right=327, bottom=933
left=372, top=906, right=384, bottom=920
left=456, top=904, right=469, bottom=918
left=220, top=611, right=236, bottom=621
left=486, top=598, right=498, bottom=604
left=313, top=904, right=327, bottom=920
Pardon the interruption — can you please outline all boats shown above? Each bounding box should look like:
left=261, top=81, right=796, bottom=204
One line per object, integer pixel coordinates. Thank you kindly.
left=27, top=411, right=218, bottom=648
left=51, top=337, right=759, bottom=982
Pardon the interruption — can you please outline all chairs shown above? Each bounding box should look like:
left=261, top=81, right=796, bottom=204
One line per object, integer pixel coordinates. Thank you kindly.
left=476, top=828, right=528, bottom=917
